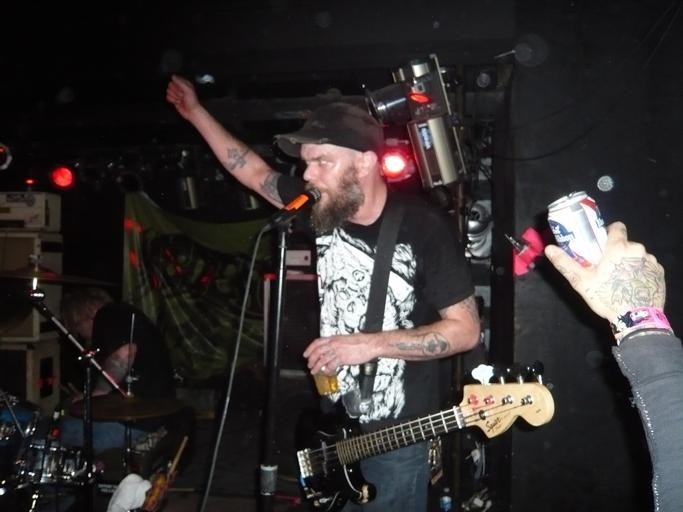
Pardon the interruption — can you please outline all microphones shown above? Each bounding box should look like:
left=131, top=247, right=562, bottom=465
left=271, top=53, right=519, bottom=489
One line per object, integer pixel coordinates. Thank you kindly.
left=268, top=188, right=322, bottom=231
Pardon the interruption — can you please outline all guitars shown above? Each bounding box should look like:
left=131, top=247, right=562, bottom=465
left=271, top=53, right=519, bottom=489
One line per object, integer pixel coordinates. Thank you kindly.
left=294, top=361, right=555, bottom=512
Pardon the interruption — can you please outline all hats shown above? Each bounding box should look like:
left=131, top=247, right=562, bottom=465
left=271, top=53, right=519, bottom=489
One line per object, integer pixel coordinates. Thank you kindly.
left=274, top=99, right=386, bottom=161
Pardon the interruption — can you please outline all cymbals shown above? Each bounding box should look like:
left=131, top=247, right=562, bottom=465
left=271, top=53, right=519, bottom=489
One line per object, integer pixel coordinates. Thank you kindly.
left=70, top=393, right=182, bottom=421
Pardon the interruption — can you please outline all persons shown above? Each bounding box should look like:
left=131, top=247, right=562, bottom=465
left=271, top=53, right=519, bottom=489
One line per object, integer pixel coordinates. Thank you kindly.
left=165, top=73, right=484, bottom=511
left=545, top=220, right=683, bottom=510
left=62, top=286, right=177, bottom=452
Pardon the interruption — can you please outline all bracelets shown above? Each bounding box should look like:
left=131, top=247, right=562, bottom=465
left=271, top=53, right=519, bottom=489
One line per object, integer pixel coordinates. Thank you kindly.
left=608, top=308, right=674, bottom=344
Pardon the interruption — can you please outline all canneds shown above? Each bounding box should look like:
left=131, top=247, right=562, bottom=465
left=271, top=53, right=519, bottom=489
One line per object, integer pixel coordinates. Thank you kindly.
left=547, top=190, right=608, bottom=266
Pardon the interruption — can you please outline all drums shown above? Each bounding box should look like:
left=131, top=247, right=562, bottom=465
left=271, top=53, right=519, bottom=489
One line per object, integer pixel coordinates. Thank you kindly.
left=8, top=442, right=95, bottom=499
left=1, top=401, right=41, bottom=440
